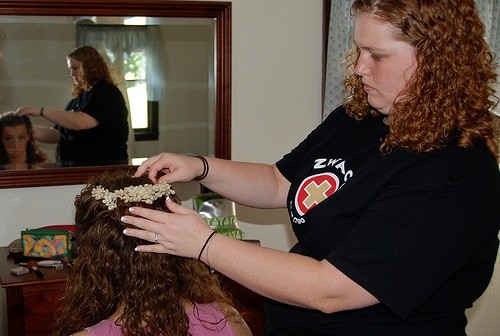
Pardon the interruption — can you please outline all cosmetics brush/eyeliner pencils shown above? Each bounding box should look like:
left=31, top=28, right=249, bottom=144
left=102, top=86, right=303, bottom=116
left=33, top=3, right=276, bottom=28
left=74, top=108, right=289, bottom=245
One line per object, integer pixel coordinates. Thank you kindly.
left=27, top=260, right=49, bottom=280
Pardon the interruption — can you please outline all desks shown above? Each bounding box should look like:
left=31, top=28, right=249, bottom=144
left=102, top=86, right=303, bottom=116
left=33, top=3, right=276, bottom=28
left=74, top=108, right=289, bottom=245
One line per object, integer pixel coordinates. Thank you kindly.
left=0, top=240, right=260, bottom=336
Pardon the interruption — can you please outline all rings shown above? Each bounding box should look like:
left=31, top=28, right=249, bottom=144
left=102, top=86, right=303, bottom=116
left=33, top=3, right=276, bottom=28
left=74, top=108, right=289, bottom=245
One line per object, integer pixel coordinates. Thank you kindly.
left=155, top=233, right=158, bottom=243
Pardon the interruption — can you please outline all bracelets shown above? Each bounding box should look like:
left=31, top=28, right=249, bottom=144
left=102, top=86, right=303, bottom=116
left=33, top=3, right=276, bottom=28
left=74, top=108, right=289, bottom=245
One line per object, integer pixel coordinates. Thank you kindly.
left=207, top=233, right=219, bottom=274
left=194, top=156, right=206, bottom=180
left=40, top=107, right=44, bottom=117
left=198, top=231, right=217, bottom=261
left=196, top=156, right=209, bottom=180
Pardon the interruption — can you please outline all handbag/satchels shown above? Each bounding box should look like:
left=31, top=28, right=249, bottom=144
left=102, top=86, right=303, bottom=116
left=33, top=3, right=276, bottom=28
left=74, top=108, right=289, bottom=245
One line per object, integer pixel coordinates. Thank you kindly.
left=20, top=227, right=75, bottom=260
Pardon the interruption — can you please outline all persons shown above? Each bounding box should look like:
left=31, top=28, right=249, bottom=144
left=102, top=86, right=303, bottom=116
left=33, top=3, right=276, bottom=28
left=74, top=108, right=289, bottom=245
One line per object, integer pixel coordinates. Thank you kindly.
left=14, top=46, right=129, bottom=166
left=0, top=112, right=55, bottom=169
left=121, top=0, right=500, bottom=336
left=59, top=167, right=254, bottom=336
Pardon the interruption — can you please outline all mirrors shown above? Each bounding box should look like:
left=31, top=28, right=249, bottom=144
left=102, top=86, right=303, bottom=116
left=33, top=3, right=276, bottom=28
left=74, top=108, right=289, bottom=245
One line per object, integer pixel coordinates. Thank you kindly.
left=0, top=0, right=232, bottom=190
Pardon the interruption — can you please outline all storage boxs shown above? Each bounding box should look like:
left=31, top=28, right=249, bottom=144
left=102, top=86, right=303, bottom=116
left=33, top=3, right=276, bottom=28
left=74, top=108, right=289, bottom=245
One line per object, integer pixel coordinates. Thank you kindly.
left=21, top=225, right=76, bottom=259
